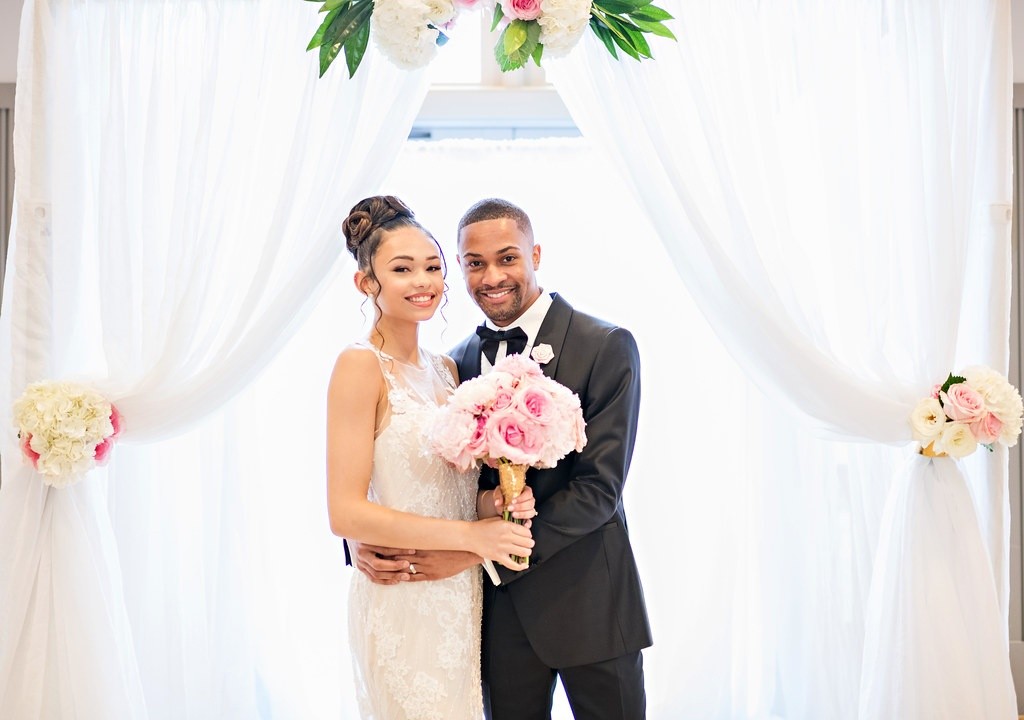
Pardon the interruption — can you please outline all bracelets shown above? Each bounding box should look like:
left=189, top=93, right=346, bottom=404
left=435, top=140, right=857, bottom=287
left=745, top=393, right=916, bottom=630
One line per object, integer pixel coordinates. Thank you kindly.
left=481, top=490, right=490, bottom=504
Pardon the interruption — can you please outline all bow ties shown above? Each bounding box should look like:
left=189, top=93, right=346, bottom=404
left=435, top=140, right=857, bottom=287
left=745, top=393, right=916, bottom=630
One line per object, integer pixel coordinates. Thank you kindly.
left=476, top=325, right=528, bottom=366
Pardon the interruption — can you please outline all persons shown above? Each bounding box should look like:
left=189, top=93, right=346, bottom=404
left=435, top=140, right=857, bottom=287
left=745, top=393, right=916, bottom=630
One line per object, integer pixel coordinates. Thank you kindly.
left=341, top=198, right=652, bottom=720
left=326, top=196, right=537, bottom=720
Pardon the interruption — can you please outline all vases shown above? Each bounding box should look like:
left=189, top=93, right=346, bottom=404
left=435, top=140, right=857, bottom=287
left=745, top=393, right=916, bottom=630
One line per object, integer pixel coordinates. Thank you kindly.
left=481, top=8, right=544, bottom=85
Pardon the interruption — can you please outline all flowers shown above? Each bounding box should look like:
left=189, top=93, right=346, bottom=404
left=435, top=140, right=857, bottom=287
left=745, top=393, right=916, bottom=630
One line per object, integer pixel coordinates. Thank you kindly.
left=305, top=0, right=679, bottom=81
left=531, top=342, right=555, bottom=363
left=13, top=380, right=124, bottom=480
left=913, top=363, right=1023, bottom=459
left=428, top=353, right=588, bottom=563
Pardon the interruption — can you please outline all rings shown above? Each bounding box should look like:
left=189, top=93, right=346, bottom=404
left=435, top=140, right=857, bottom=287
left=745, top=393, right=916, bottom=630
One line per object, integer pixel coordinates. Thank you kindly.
left=410, top=565, right=416, bottom=574
